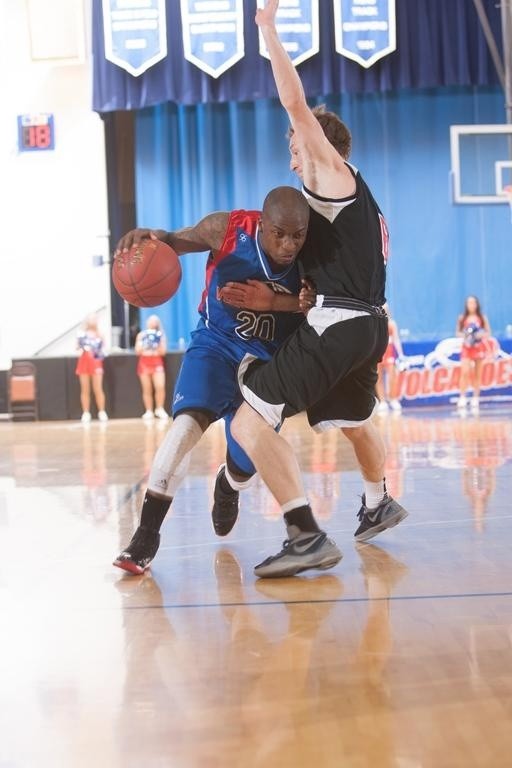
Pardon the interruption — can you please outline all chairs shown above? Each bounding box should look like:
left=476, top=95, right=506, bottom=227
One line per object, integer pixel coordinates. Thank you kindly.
left=6, top=362, right=39, bottom=422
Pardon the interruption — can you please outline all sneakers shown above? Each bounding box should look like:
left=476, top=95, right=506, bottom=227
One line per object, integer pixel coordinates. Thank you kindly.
left=253, top=524, right=345, bottom=580
left=470, top=397, right=480, bottom=408
left=155, top=408, right=169, bottom=420
left=389, top=400, right=402, bottom=411
left=141, top=410, right=154, bottom=421
left=376, top=401, right=389, bottom=412
left=97, top=411, right=108, bottom=423
left=210, top=462, right=241, bottom=538
left=456, top=396, right=468, bottom=408
left=112, top=526, right=161, bottom=577
left=353, top=489, right=409, bottom=544
left=79, top=412, right=92, bottom=424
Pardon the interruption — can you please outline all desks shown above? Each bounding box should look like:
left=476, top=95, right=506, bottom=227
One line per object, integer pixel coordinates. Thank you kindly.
left=12, top=351, right=195, bottom=419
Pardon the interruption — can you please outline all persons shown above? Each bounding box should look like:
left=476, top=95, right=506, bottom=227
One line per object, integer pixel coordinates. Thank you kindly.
left=456, top=296, right=490, bottom=406
left=384, top=448, right=401, bottom=501
left=76, top=315, right=108, bottom=422
left=309, top=427, right=337, bottom=517
left=113, top=186, right=309, bottom=575
left=461, top=457, right=496, bottom=532
left=253, top=0, right=410, bottom=579
left=374, top=304, right=405, bottom=415
left=134, top=315, right=170, bottom=420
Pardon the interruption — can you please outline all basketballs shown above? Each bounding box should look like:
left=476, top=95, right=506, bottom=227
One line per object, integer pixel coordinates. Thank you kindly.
left=112, top=241, right=180, bottom=308
